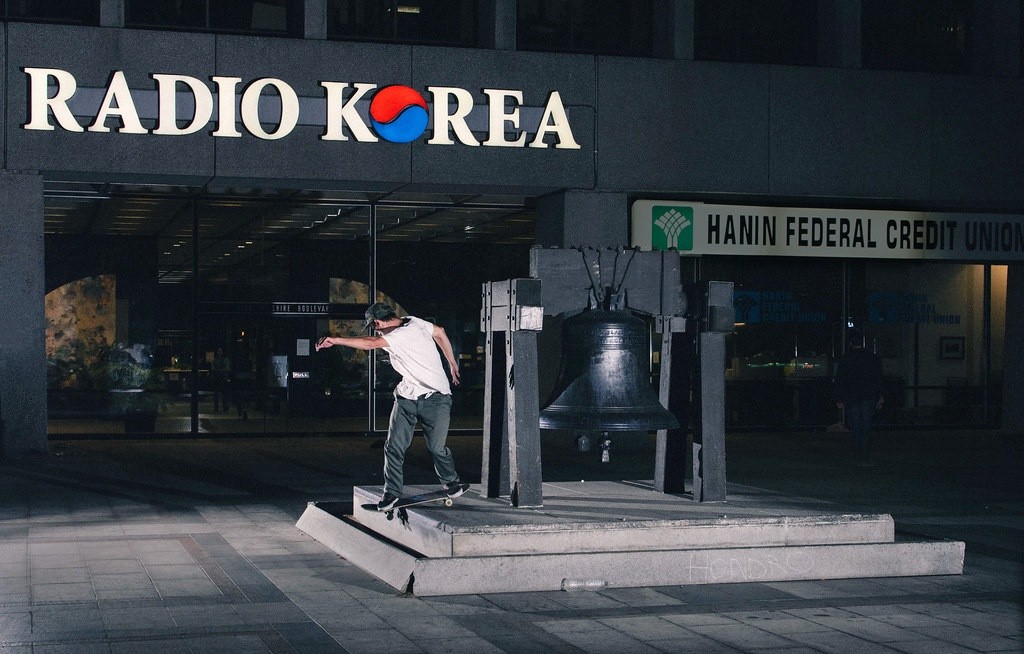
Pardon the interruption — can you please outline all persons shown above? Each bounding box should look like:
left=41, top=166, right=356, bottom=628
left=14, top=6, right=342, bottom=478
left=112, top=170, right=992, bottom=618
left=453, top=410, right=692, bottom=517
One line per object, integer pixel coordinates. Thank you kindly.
left=837, top=333, right=886, bottom=470
left=314, top=303, right=463, bottom=511
left=210, top=346, right=229, bottom=413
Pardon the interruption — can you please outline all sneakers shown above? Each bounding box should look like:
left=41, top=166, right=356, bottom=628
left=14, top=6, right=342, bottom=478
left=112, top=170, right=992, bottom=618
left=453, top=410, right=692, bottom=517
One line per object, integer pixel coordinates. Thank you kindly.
left=377, top=492, right=400, bottom=513
left=446, top=480, right=464, bottom=500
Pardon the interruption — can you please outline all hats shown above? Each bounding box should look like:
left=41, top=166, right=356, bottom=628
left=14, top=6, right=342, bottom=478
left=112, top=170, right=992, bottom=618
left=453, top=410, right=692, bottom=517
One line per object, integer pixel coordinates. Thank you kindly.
left=360, top=302, right=395, bottom=332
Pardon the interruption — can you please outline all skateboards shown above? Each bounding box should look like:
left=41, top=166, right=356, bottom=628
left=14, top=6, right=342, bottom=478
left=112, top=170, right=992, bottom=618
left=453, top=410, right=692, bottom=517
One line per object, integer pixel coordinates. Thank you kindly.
left=361, top=485, right=471, bottom=520
